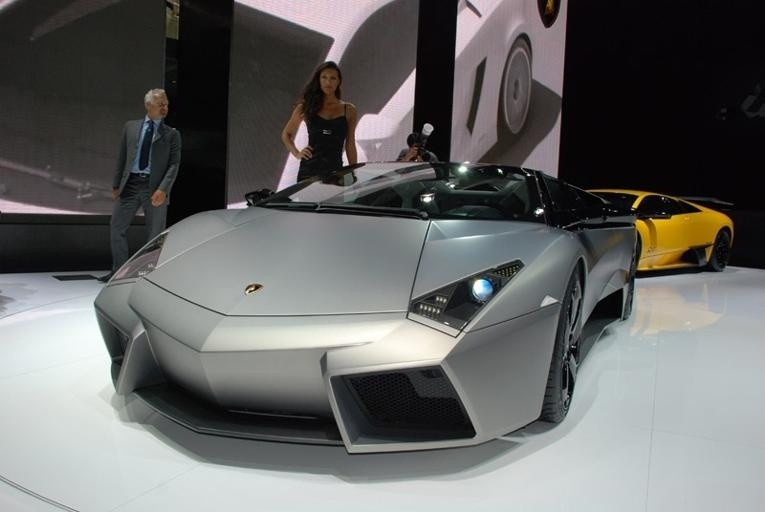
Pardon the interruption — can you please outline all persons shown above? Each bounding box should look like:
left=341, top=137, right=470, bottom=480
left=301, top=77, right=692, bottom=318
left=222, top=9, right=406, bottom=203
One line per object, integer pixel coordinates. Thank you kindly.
left=99, top=89, right=181, bottom=282
left=395, top=133, right=440, bottom=162
left=280, top=61, right=358, bottom=183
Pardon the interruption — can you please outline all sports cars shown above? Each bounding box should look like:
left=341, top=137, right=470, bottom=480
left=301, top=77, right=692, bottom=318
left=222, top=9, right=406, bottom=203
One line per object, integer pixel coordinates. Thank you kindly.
left=1, top=0, right=563, bottom=217
left=94, top=160, right=638, bottom=454
left=585, top=189, right=736, bottom=273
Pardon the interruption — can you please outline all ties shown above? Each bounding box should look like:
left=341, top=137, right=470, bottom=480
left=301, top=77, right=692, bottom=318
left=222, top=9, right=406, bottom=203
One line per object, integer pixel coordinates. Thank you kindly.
left=139, top=120, right=155, bottom=170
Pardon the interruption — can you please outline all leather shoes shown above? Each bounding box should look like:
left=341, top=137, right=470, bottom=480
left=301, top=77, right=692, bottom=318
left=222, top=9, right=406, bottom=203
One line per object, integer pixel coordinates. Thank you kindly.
left=98, top=272, right=114, bottom=281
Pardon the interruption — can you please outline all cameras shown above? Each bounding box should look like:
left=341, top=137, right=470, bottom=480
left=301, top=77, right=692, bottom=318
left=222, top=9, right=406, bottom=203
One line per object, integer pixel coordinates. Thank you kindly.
left=413, top=145, right=431, bottom=161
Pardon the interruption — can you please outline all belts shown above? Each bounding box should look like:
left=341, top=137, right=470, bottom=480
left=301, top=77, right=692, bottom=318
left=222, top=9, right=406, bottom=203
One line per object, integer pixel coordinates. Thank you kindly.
left=130, top=173, right=150, bottom=178
